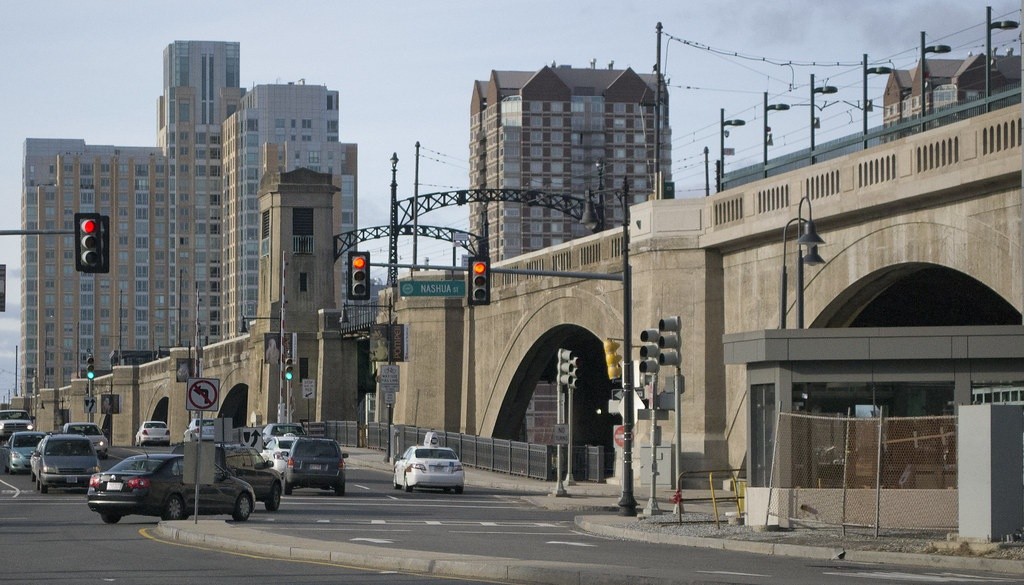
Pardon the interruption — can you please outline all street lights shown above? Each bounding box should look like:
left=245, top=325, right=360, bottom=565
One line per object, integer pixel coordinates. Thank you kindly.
left=920, top=31, right=951, bottom=132
left=862, top=53, right=892, bottom=149
left=779, top=195, right=824, bottom=329
left=763, top=92, right=790, bottom=178
left=985, top=6, right=1020, bottom=114
left=719, top=107, right=746, bottom=191
left=810, top=73, right=837, bottom=164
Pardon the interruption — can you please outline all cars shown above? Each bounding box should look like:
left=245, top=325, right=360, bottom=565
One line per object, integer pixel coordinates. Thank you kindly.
left=135, top=419, right=214, bottom=446
left=87, top=453, right=256, bottom=524
left=0, top=410, right=109, bottom=494
left=393, top=431, right=464, bottom=493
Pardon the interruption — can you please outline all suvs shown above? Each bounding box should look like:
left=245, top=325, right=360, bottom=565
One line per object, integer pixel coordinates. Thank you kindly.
left=261, top=423, right=349, bottom=496
left=172, top=445, right=283, bottom=511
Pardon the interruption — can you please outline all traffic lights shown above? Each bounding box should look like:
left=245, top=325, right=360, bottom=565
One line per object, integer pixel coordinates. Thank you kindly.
left=347, top=251, right=370, bottom=301
left=75, top=212, right=102, bottom=272
left=557, top=349, right=580, bottom=390
left=604, top=338, right=623, bottom=379
left=86, top=357, right=94, bottom=379
left=468, top=257, right=490, bottom=305
left=639, top=314, right=682, bottom=374
left=284, top=358, right=293, bottom=380
